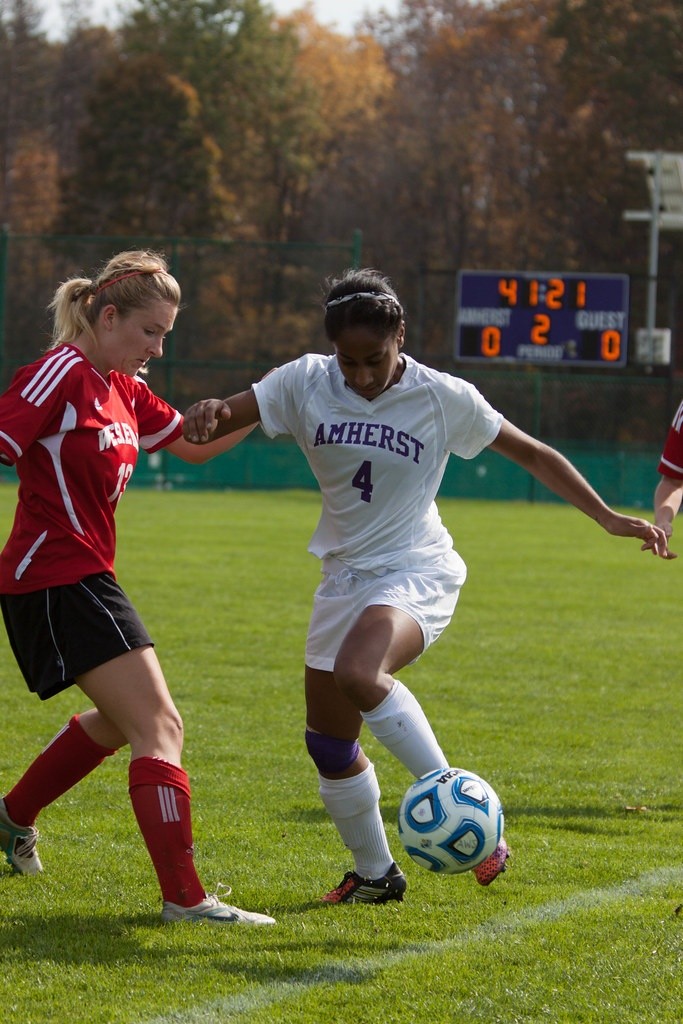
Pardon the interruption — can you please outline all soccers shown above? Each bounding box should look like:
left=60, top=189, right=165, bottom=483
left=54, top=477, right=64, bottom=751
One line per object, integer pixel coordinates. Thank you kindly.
left=398, top=767, right=505, bottom=874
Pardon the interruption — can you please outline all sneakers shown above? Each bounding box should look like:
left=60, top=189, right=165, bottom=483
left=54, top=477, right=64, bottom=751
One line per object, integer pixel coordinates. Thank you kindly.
left=321, top=860, right=408, bottom=903
left=470, top=836, right=510, bottom=886
left=159, top=882, right=276, bottom=926
left=0, top=797, right=44, bottom=876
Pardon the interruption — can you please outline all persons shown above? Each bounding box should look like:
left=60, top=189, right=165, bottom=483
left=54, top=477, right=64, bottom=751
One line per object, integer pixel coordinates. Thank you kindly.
left=182, top=265, right=669, bottom=904
left=654, top=401, right=683, bottom=560
left=1, top=251, right=276, bottom=927
